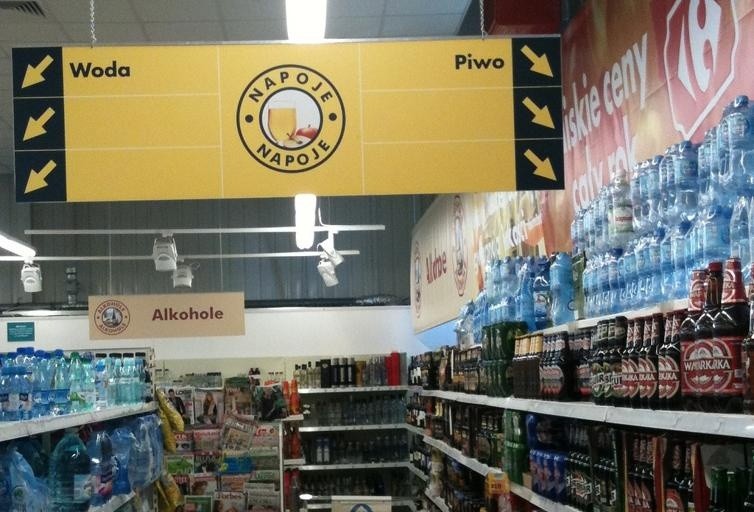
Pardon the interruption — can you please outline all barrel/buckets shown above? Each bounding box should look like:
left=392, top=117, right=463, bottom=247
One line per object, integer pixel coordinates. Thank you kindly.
left=0, top=414, right=160, bottom=512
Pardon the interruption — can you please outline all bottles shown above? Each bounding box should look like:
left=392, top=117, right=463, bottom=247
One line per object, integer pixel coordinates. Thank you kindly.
left=407, top=94, right=754, bottom=511
left=0, top=346, right=163, bottom=511
left=155, top=368, right=286, bottom=388
left=284, top=353, right=407, bottom=511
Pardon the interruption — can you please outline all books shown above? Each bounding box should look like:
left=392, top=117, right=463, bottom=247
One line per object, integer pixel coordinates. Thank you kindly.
left=159, top=375, right=281, bottom=512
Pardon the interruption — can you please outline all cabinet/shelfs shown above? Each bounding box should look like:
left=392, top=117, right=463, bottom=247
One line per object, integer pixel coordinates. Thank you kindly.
left=279, top=285, right=753, bottom=512
left=0, top=355, right=159, bottom=512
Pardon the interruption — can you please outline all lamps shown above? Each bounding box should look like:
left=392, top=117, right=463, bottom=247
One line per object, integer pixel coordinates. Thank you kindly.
left=313, top=229, right=344, bottom=287
left=146, top=230, right=197, bottom=290
left=0, top=232, right=45, bottom=295
